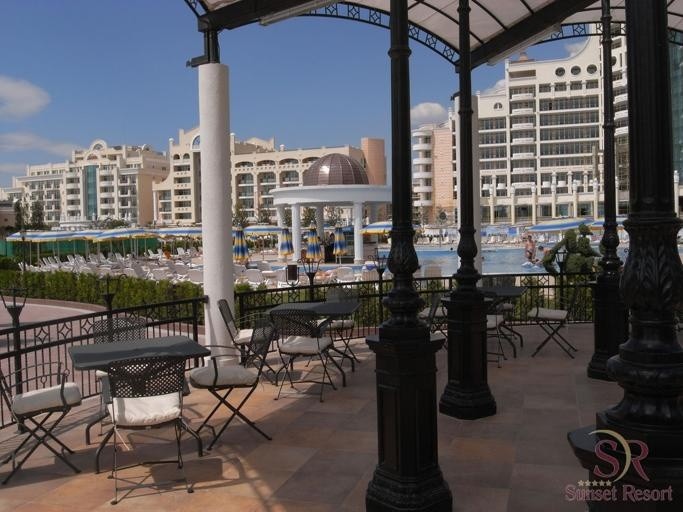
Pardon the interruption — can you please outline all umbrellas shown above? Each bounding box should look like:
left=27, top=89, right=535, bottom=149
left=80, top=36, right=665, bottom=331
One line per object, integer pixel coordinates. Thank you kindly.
left=5, top=222, right=292, bottom=275
left=527, top=215, right=594, bottom=239
left=615, top=216, right=628, bottom=241
left=591, top=220, right=605, bottom=235
left=358, top=220, right=421, bottom=249
left=305, top=220, right=324, bottom=265
left=333, top=221, right=352, bottom=264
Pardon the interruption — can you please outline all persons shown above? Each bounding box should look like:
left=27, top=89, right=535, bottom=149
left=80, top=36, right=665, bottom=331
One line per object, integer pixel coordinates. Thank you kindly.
left=522, top=236, right=553, bottom=269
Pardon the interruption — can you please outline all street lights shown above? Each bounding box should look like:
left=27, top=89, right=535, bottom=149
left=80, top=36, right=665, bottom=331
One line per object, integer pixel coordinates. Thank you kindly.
left=295, top=256, right=326, bottom=301
left=0, top=281, right=30, bottom=434
left=19, top=228, right=27, bottom=270
left=95, top=273, right=120, bottom=342
left=367, top=254, right=388, bottom=325
